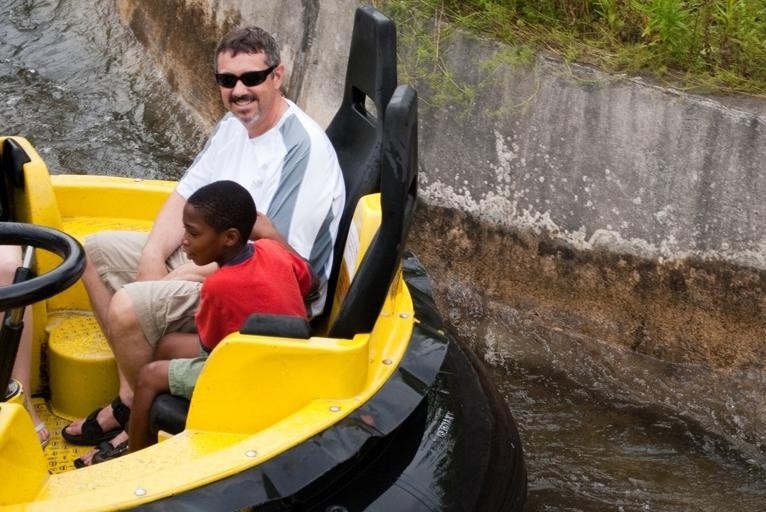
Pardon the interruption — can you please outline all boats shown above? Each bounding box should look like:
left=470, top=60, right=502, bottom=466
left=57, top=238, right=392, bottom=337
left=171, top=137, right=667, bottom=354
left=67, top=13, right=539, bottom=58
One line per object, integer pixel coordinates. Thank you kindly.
left=0, top=5, right=526, bottom=512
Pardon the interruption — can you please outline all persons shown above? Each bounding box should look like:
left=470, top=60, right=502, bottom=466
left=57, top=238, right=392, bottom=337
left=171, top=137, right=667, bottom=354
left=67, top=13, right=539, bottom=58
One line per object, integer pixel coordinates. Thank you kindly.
left=0, top=245, right=49, bottom=448
left=128, top=180, right=321, bottom=454
left=62, top=26, right=346, bottom=468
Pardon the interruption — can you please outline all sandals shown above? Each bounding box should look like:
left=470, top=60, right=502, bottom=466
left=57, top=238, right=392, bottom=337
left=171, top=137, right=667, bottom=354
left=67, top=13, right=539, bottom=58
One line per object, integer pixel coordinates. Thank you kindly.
left=75, top=423, right=131, bottom=469
left=63, top=396, right=131, bottom=444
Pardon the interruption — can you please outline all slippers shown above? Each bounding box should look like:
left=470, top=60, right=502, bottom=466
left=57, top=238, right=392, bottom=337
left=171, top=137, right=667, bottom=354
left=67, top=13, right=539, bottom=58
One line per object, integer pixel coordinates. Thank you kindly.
left=35, top=421, right=49, bottom=449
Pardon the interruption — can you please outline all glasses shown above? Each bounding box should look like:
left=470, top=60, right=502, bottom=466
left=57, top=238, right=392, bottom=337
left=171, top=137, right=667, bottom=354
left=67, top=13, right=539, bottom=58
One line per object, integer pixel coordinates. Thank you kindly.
left=214, top=66, right=276, bottom=86
